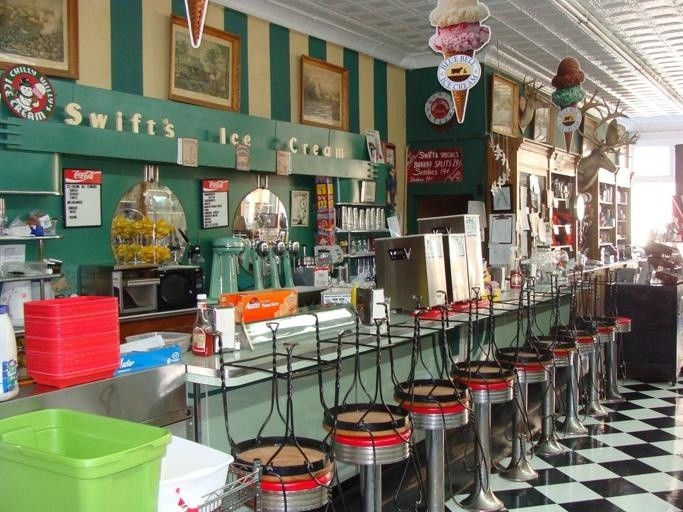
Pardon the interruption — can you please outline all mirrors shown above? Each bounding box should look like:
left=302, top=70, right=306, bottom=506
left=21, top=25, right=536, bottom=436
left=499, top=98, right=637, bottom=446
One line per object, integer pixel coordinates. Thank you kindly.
left=109, top=181, right=188, bottom=266
left=231, top=188, right=290, bottom=278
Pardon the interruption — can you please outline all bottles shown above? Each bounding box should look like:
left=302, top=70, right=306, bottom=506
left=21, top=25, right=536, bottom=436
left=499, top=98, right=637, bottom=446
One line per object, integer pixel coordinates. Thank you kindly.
left=509, top=258, right=523, bottom=289
left=192, top=294, right=213, bottom=357
left=194, top=250, right=206, bottom=294
left=235, top=332, right=240, bottom=351
left=339, top=237, right=369, bottom=254
left=0, top=305, right=20, bottom=401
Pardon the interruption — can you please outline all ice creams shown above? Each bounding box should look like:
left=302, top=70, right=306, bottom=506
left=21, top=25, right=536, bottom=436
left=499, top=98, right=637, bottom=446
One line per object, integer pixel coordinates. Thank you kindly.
left=551, top=57, right=585, bottom=156
left=428, top=0, right=491, bottom=123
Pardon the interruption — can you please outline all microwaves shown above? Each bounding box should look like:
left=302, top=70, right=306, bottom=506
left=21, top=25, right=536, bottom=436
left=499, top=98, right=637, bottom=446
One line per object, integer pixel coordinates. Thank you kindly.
left=154, top=265, right=204, bottom=312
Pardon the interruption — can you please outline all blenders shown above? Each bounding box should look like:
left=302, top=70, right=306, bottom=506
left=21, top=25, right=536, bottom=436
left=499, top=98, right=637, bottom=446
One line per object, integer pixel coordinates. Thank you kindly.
left=314, top=244, right=348, bottom=288
left=209, top=237, right=246, bottom=302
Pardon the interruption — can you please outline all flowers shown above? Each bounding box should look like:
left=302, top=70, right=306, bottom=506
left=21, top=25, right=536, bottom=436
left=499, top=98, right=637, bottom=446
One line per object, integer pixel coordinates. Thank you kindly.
left=111, top=215, right=176, bottom=265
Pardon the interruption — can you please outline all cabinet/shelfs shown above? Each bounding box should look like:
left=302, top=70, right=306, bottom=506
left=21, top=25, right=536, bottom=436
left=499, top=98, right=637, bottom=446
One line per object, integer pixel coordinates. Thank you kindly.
left=487, top=137, right=557, bottom=265
left=617, top=168, right=635, bottom=261
left=582, top=165, right=621, bottom=262
left=550, top=147, right=583, bottom=265
left=567, top=257, right=638, bottom=322
left=604, top=284, right=683, bottom=387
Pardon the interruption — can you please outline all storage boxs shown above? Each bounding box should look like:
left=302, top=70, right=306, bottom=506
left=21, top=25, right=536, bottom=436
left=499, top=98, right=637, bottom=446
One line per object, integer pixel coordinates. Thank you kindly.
left=0, top=407, right=172, bottom=512
left=159, top=434, right=236, bottom=512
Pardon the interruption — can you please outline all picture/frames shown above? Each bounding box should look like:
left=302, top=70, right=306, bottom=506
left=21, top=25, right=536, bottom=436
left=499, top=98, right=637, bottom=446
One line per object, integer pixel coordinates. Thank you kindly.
left=301, top=54, right=351, bottom=132
left=486, top=71, right=521, bottom=138
left=528, top=104, right=556, bottom=145
left=0, top=0, right=82, bottom=81
left=168, top=13, right=242, bottom=115
left=580, top=115, right=598, bottom=158
